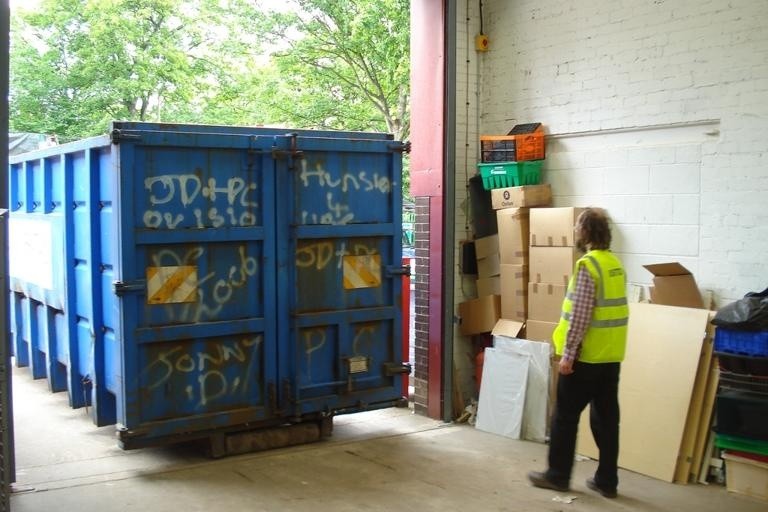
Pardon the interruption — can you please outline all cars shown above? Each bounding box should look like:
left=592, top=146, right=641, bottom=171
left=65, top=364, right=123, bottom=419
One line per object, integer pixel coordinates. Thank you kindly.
left=401, top=221, right=415, bottom=260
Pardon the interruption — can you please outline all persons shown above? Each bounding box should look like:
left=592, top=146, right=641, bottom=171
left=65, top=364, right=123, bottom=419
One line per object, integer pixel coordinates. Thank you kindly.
left=528, top=209, right=629, bottom=499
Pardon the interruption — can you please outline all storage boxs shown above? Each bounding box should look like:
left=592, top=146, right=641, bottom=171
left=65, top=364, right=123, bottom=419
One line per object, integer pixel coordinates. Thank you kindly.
left=710, top=326, right=768, bottom=503
left=456, top=185, right=601, bottom=442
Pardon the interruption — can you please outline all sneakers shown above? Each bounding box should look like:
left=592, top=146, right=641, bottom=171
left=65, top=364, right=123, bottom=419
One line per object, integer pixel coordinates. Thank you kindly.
left=586, top=476, right=618, bottom=499
left=527, top=470, right=569, bottom=491
